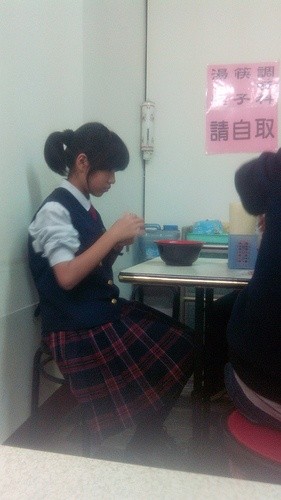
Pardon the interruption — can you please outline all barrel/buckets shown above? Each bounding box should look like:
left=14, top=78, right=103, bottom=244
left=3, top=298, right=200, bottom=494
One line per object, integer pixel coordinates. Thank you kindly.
left=138, top=223, right=180, bottom=264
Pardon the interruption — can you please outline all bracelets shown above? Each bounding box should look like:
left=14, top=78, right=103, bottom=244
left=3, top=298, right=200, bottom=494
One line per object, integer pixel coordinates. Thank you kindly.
left=111, top=250, right=123, bottom=256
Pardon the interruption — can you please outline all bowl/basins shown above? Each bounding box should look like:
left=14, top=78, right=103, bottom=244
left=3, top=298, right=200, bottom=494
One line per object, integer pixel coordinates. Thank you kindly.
left=186, top=233, right=229, bottom=246
left=155, top=239, right=208, bottom=267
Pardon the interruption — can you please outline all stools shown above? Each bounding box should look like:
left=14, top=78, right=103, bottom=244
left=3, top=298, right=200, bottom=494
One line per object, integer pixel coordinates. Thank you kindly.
left=30, top=339, right=98, bottom=457
left=211, top=405, right=281, bottom=484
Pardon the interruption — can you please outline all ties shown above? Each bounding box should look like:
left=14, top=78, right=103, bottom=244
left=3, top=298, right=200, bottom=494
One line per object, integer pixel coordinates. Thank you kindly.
left=87, top=206, right=97, bottom=223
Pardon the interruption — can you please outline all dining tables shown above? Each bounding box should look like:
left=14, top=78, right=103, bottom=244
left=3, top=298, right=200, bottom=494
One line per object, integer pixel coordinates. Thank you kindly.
left=118, top=253, right=262, bottom=471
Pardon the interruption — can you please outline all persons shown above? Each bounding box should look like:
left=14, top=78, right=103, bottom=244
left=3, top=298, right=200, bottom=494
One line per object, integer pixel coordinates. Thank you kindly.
left=222, top=147, right=281, bottom=427
left=192, top=288, right=238, bottom=401
left=29, top=122, right=202, bottom=462
